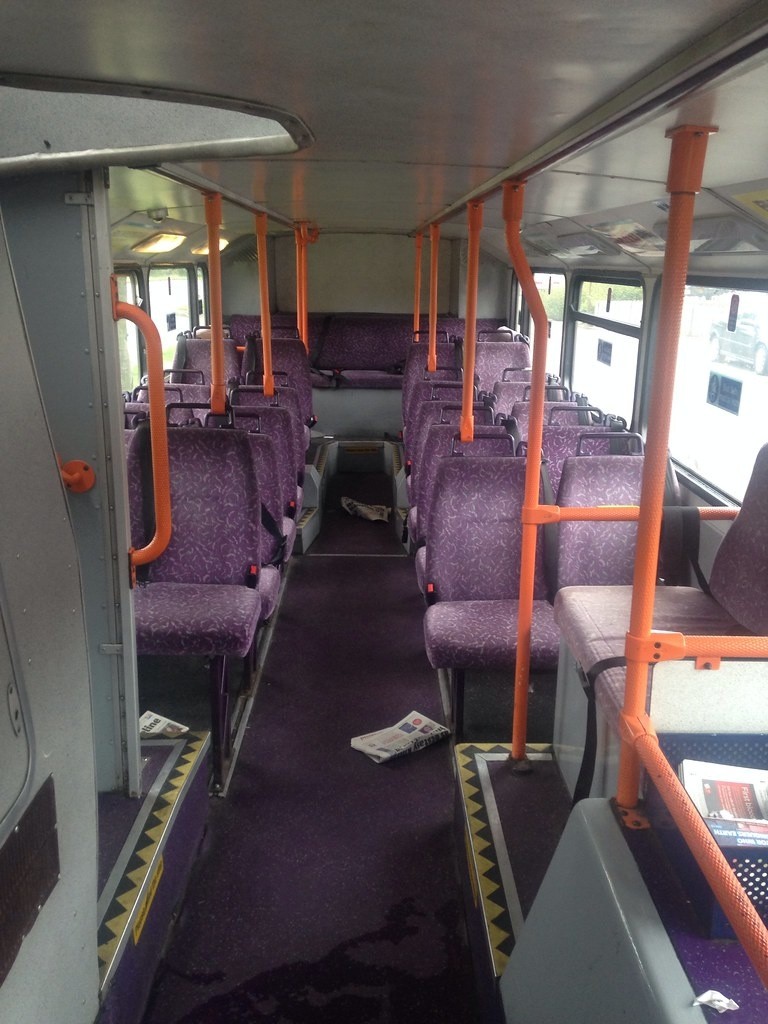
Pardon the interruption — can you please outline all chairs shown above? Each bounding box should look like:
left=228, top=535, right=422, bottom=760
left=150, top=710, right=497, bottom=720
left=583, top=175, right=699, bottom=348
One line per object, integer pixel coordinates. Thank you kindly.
left=400, top=328, right=685, bottom=744
left=120, top=314, right=321, bottom=797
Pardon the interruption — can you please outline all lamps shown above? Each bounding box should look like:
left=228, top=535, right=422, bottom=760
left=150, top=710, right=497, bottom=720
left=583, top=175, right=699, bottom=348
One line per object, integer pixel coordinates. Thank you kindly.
left=191, top=237, right=229, bottom=256
left=129, top=232, right=185, bottom=255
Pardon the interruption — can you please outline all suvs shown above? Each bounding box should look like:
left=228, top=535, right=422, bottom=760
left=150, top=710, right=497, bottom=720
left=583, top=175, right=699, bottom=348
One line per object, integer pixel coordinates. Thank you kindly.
left=708, top=316, right=767, bottom=374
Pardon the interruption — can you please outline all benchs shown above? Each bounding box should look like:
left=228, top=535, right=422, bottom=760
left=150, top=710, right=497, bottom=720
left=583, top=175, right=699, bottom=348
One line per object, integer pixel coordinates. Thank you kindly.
left=545, top=443, right=768, bottom=812
left=229, top=311, right=507, bottom=390
